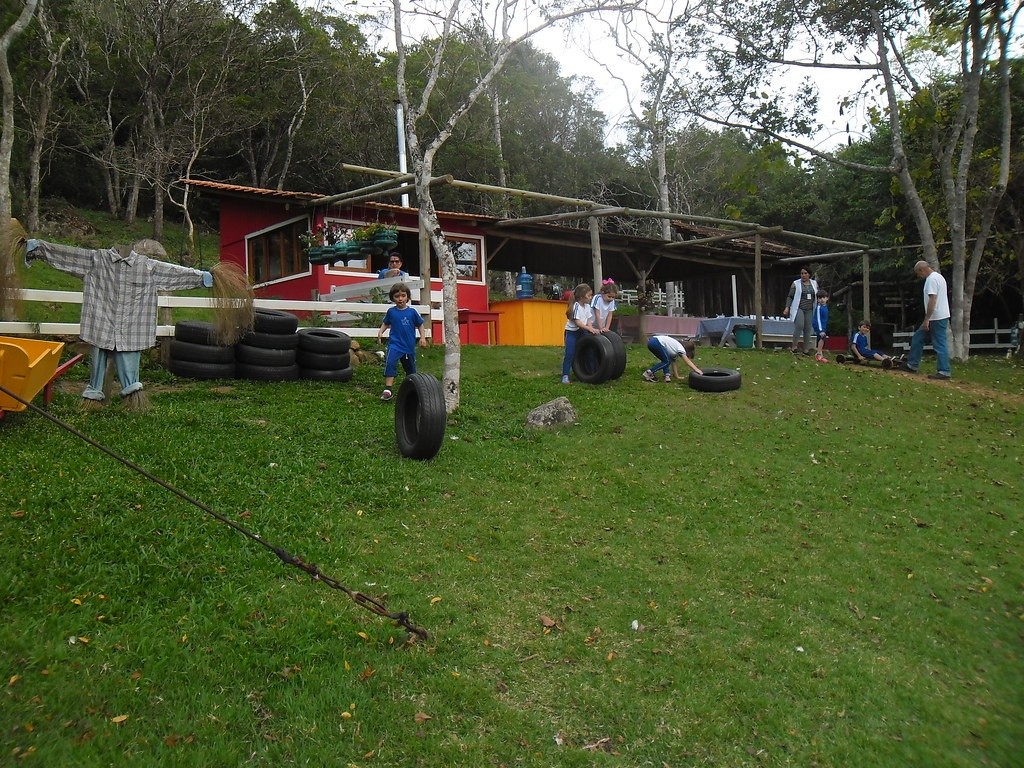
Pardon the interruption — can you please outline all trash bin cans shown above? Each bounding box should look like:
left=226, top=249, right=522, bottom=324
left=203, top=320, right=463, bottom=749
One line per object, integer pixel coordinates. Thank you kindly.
left=733, top=324, right=757, bottom=349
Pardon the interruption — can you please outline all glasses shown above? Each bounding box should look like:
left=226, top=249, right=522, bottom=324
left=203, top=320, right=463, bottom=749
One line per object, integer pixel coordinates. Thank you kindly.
left=390, top=260, right=401, bottom=264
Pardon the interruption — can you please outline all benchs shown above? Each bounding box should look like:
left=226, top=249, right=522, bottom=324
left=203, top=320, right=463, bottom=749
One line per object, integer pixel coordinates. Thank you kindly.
left=644, top=332, right=695, bottom=337
left=729, top=333, right=829, bottom=348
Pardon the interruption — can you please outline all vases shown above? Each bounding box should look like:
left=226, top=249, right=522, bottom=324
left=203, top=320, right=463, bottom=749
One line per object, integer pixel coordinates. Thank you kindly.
left=309, top=230, right=398, bottom=266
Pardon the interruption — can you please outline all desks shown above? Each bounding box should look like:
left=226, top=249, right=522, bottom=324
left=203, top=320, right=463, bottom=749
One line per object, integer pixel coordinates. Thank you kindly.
left=432, top=310, right=505, bottom=345
left=696, top=318, right=818, bottom=348
left=617, top=313, right=708, bottom=344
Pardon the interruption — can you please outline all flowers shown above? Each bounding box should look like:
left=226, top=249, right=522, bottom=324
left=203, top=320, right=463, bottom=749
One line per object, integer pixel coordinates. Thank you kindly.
left=298, top=216, right=398, bottom=253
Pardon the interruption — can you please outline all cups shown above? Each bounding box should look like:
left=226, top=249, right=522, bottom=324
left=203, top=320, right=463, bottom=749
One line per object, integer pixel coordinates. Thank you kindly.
left=750, top=315, right=780, bottom=321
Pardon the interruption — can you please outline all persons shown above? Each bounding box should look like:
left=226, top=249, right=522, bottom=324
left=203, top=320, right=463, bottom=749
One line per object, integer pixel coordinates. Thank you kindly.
left=590, top=278, right=618, bottom=332
left=544, top=281, right=559, bottom=300
left=783, top=266, right=820, bottom=357
left=643, top=335, right=703, bottom=382
left=377, top=283, right=426, bottom=400
left=899, top=261, right=951, bottom=381
left=562, top=284, right=600, bottom=384
left=812, top=290, right=830, bottom=363
left=850, top=320, right=905, bottom=361
left=378, top=252, right=406, bottom=279
left=1015, top=314, right=1024, bottom=350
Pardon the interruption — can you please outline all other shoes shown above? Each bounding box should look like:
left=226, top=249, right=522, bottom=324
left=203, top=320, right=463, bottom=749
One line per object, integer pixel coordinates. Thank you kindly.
left=928, top=372, right=951, bottom=379
left=821, top=358, right=829, bottom=363
left=803, top=351, right=811, bottom=357
left=643, top=371, right=659, bottom=382
left=816, top=354, right=822, bottom=361
left=790, top=349, right=800, bottom=355
left=665, top=376, right=672, bottom=382
left=899, top=364, right=917, bottom=373
left=381, top=390, right=393, bottom=400
left=899, top=354, right=906, bottom=360
left=888, top=356, right=897, bottom=361
left=561, top=375, right=570, bottom=383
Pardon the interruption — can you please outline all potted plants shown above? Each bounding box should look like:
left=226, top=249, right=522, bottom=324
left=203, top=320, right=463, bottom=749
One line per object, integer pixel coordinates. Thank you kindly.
left=631, top=297, right=667, bottom=315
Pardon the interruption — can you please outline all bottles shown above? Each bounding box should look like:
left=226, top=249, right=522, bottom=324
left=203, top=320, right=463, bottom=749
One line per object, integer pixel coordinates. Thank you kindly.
left=515, top=266, right=534, bottom=299
